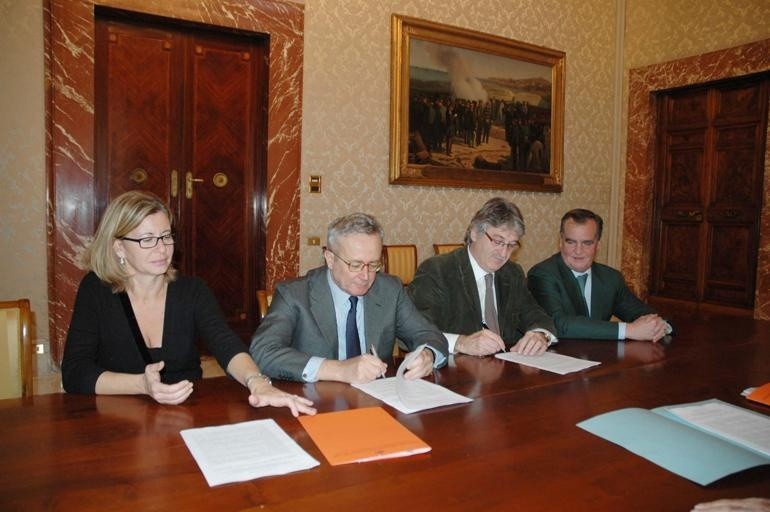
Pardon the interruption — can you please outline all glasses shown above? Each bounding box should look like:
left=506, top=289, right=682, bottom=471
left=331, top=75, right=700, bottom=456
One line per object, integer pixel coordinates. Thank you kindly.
left=116, top=233, right=179, bottom=249
left=329, top=248, right=382, bottom=272
left=485, top=232, right=520, bottom=251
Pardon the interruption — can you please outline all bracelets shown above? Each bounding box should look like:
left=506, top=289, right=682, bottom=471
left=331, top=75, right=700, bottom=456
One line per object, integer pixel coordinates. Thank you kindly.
left=246, top=373, right=272, bottom=387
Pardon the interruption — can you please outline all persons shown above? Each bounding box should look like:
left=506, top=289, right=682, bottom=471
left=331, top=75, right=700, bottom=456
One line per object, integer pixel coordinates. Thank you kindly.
left=527, top=207, right=673, bottom=344
left=408, top=95, right=551, bottom=174
left=397, top=197, right=561, bottom=357
left=688, top=494, right=769, bottom=512
left=62, top=189, right=317, bottom=419
left=251, top=212, right=448, bottom=384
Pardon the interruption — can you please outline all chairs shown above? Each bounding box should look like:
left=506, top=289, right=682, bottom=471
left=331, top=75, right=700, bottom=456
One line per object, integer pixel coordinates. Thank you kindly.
left=253, top=286, right=277, bottom=322
left=0, top=296, right=42, bottom=407
left=432, top=242, right=468, bottom=257
left=381, top=242, right=421, bottom=287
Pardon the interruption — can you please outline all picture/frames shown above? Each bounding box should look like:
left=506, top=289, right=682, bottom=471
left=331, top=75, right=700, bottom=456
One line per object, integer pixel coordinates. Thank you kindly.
left=386, top=11, right=568, bottom=194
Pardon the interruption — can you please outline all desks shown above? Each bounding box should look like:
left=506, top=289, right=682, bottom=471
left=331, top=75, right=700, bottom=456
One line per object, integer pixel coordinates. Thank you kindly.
left=0, top=317, right=769, bottom=512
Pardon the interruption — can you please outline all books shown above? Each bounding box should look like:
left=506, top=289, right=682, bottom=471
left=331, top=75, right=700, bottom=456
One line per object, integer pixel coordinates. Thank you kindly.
left=574, top=395, right=766, bottom=487
left=289, top=406, right=433, bottom=468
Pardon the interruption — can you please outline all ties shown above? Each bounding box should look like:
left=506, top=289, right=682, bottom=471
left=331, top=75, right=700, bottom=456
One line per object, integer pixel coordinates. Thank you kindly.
left=484, top=274, right=499, bottom=335
left=576, top=274, right=589, bottom=298
left=346, top=296, right=361, bottom=360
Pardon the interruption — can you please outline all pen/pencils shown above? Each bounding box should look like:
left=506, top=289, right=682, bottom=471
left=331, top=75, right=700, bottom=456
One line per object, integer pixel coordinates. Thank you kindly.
left=370, top=343, right=386, bottom=378
left=481, top=321, right=507, bottom=354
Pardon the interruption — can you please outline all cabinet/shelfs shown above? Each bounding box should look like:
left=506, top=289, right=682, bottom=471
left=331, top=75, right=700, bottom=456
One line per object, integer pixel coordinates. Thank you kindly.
left=647, top=69, right=769, bottom=309
